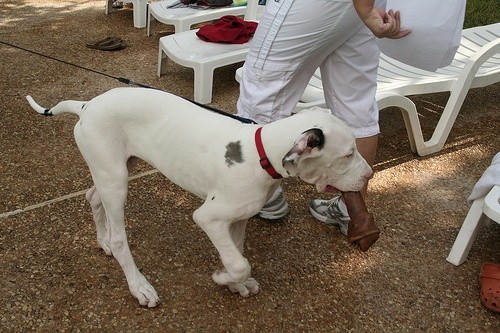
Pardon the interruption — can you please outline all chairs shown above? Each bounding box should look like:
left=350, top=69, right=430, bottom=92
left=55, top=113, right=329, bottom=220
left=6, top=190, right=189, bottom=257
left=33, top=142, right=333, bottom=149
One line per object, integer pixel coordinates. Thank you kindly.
left=445, top=151, right=500, bottom=266
left=156, top=0, right=259, bottom=104
left=105, top=0, right=156, bottom=28
left=147, top=0, right=258, bottom=37
left=236, top=20, right=500, bottom=157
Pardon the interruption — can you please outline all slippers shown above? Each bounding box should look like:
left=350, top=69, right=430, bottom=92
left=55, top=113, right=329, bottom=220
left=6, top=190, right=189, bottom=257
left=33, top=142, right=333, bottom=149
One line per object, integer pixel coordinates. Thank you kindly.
left=479, top=262, right=500, bottom=314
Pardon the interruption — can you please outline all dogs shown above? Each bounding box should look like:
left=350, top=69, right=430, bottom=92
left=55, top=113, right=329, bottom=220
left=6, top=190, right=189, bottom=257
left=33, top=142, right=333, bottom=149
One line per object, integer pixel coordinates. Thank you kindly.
left=24, top=84, right=375, bottom=309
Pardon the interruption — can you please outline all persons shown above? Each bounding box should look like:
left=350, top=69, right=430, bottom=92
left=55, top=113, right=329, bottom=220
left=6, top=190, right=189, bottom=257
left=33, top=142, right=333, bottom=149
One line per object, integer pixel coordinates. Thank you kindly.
left=232, top=0, right=413, bottom=236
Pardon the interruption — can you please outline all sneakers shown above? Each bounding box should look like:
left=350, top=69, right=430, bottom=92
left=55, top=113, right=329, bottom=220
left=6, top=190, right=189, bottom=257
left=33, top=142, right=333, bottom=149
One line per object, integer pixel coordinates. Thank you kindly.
left=257, top=186, right=291, bottom=219
left=309, top=195, right=350, bottom=235
